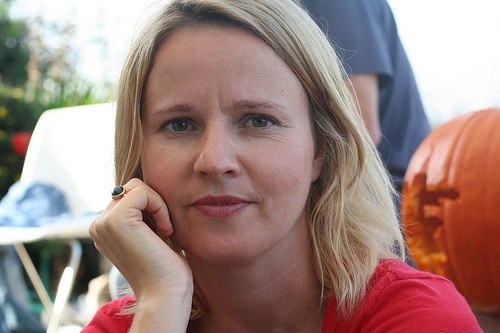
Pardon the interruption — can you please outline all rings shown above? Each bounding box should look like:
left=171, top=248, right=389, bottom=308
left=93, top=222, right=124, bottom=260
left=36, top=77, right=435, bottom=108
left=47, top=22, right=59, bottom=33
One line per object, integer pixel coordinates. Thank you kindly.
left=111, top=186, right=127, bottom=200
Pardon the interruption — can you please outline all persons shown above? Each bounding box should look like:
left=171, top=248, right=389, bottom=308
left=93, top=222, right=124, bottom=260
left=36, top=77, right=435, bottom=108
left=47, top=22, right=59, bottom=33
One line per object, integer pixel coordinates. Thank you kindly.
left=80, top=0, right=483, bottom=333
left=295, top=0, right=431, bottom=274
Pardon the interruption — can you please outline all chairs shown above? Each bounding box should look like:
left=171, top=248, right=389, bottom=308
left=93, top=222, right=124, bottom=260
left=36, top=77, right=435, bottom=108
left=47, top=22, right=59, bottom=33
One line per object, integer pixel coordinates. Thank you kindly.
left=0, top=99, right=116, bottom=333
